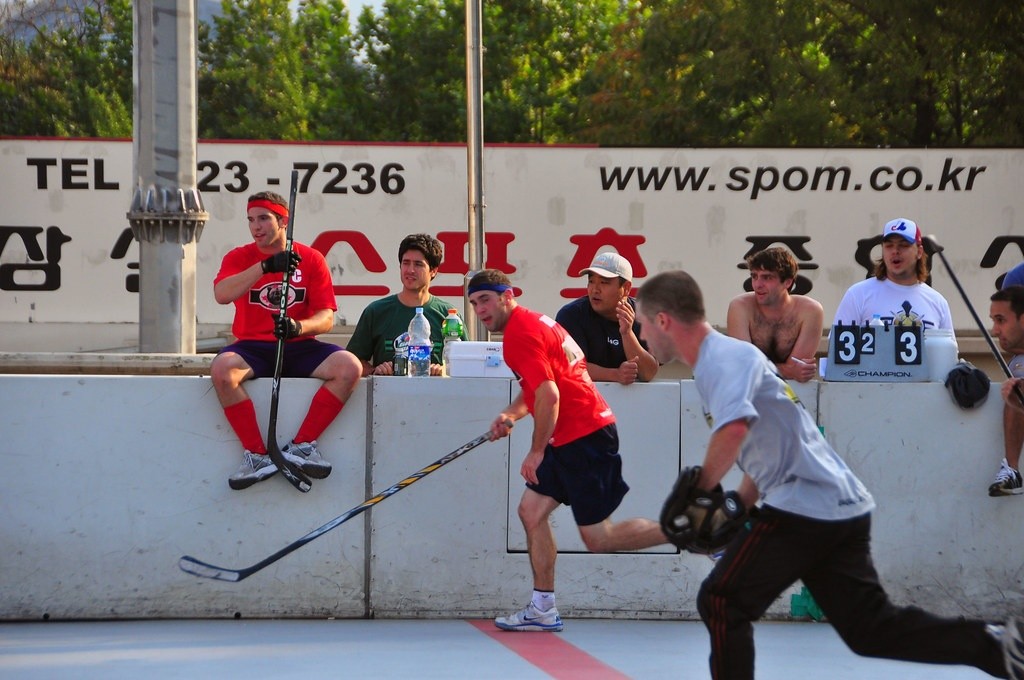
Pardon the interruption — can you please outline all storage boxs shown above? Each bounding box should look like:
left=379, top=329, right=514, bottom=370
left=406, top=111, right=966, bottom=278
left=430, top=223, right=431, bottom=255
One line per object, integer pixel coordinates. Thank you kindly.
left=444, top=342, right=515, bottom=377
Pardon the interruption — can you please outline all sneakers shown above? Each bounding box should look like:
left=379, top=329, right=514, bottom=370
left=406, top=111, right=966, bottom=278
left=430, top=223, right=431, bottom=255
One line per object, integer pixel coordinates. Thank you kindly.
left=989, top=613, right=1024, bottom=680
left=279, top=439, right=332, bottom=480
left=227, top=450, right=278, bottom=491
left=494, top=602, right=563, bottom=632
left=988, top=457, right=1023, bottom=495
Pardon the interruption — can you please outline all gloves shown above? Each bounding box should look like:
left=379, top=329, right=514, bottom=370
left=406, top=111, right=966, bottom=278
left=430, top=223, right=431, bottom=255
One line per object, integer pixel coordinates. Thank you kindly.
left=261, top=251, right=301, bottom=275
left=272, top=312, right=302, bottom=340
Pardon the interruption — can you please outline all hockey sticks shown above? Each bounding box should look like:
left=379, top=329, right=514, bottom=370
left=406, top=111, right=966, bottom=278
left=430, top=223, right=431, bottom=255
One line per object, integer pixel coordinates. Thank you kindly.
left=178, top=417, right=514, bottom=584
left=264, top=168, right=315, bottom=494
left=922, top=233, right=1024, bottom=406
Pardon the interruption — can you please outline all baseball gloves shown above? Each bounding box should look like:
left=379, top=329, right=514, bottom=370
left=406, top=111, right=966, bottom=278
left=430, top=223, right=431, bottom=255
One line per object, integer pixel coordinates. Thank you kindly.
left=659, top=464, right=747, bottom=558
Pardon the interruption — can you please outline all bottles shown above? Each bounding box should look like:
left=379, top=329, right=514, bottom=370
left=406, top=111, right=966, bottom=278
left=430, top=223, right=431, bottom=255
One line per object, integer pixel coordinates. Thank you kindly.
left=408, top=307, right=432, bottom=377
left=924, top=328, right=959, bottom=383
left=441, top=309, right=464, bottom=376
left=870, top=314, right=884, bottom=326
left=393, top=348, right=407, bottom=376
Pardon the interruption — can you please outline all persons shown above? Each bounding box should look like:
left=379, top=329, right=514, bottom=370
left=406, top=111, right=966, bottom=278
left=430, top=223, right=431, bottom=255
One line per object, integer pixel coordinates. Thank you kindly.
left=208, top=192, right=363, bottom=490
left=634, top=271, right=1024, bottom=680
left=466, top=269, right=727, bottom=631
left=726, top=248, right=825, bottom=383
left=827, top=219, right=960, bottom=379
left=346, top=233, right=468, bottom=377
left=988, top=264, right=1024, bottom=496
left=555, top=254, right=659, bottom=382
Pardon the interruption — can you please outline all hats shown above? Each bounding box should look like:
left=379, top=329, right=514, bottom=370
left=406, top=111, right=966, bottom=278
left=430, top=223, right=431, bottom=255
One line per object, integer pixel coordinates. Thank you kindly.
left=883, top=218, right=921, bottom=244
left=579, top=252, right=633, bottom=283
